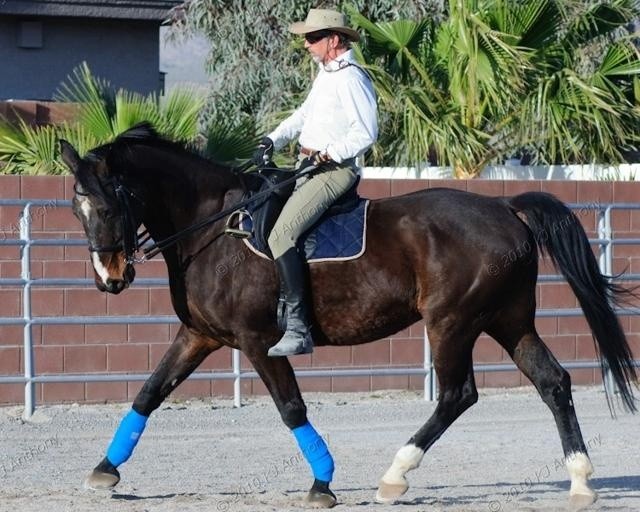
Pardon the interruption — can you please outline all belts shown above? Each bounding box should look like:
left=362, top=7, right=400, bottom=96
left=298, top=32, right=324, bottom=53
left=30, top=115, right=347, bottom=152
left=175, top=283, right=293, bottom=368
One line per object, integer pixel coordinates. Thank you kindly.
left=300, top=148, right=313, bottom=154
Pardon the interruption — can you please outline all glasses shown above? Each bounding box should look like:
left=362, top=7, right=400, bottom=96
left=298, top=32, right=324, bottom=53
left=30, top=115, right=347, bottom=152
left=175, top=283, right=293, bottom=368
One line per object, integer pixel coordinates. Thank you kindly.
left=306, top=31, right=332, bottom=43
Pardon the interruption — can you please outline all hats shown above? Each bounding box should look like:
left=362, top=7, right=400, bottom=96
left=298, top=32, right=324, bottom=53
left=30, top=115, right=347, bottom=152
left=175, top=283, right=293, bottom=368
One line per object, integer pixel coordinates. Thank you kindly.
left=288, top=9, right=360, bottom=43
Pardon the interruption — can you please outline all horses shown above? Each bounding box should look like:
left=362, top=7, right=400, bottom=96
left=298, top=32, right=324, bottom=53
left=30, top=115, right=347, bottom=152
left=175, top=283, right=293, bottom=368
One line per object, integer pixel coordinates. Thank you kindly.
left=54, top=119, right=640, bottom=509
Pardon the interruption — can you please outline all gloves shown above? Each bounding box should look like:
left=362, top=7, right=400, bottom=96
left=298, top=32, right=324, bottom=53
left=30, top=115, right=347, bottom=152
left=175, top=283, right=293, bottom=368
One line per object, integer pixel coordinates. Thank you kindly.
left=254, top=138, right=272, bottom=166
left=298, top=152, right=325, bottom=179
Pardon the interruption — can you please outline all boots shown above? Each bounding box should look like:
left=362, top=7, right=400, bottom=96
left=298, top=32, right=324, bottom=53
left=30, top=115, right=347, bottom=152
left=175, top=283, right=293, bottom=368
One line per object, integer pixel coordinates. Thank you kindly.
left=268, top=245, right=312, bottom=357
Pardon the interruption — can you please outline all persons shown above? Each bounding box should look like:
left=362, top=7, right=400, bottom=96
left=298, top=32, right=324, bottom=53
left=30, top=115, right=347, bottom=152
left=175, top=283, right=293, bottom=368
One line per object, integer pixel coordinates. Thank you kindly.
left=251, top=9, right=379, bottom=355
